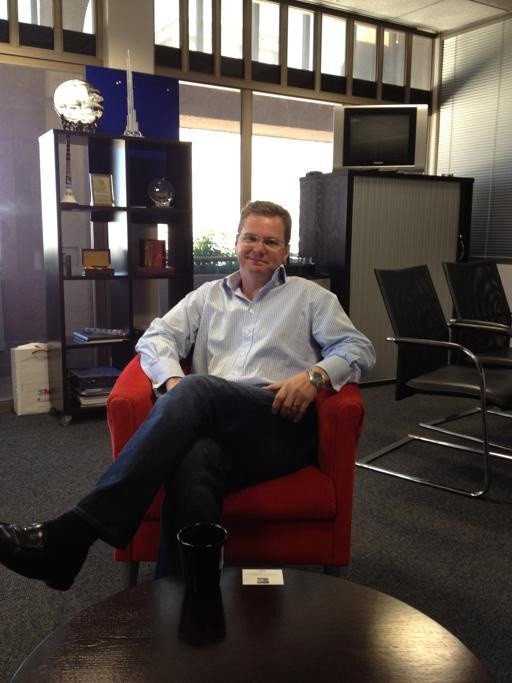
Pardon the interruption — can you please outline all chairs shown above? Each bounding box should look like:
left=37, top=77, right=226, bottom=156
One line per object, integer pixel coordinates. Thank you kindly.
left=100, top=349, right=367, bottom=591
left=354, top=264, right=511, bottom=504
left=416, top=261, right=511, bottom=454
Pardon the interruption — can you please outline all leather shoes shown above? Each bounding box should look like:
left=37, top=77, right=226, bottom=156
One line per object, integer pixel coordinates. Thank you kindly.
left=0, top=511, right=96, bottom=594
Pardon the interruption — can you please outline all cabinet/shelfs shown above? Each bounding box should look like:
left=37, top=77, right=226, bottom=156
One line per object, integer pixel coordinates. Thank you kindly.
left=296, top=164, right=477, bottom=394
left=294, top=270, right=335, bottom=295
left=37, top=129, right=195, bottom=429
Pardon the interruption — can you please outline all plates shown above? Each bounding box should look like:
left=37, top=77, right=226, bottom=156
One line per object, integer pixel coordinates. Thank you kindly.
left=141, top=175, right=178, bottom=208
left=55, top=78, right=105, bottom=123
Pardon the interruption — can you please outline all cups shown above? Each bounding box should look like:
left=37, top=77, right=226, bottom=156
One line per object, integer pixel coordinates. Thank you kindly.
left=177, top=519, right=228, bottom=588
left=177, top=581, right=226, bottom=650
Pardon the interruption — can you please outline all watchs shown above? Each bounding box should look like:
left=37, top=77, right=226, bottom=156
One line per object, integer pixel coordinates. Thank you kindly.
left=306, top=367, right=325, bottom=392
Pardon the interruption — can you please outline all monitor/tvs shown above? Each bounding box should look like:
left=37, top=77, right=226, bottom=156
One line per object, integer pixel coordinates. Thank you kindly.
left=332, top=104, right=429, bottom=171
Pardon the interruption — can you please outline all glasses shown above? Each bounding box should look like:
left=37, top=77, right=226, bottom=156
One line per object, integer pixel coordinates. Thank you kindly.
left=238, top=234, right=286, bottom=245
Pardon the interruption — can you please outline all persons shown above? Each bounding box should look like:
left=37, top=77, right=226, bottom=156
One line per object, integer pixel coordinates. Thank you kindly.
left=0, top=201, right=376, bottom=591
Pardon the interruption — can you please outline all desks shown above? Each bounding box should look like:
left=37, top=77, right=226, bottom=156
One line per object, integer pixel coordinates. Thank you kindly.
left=6, top=564, right=491, bottom=680
